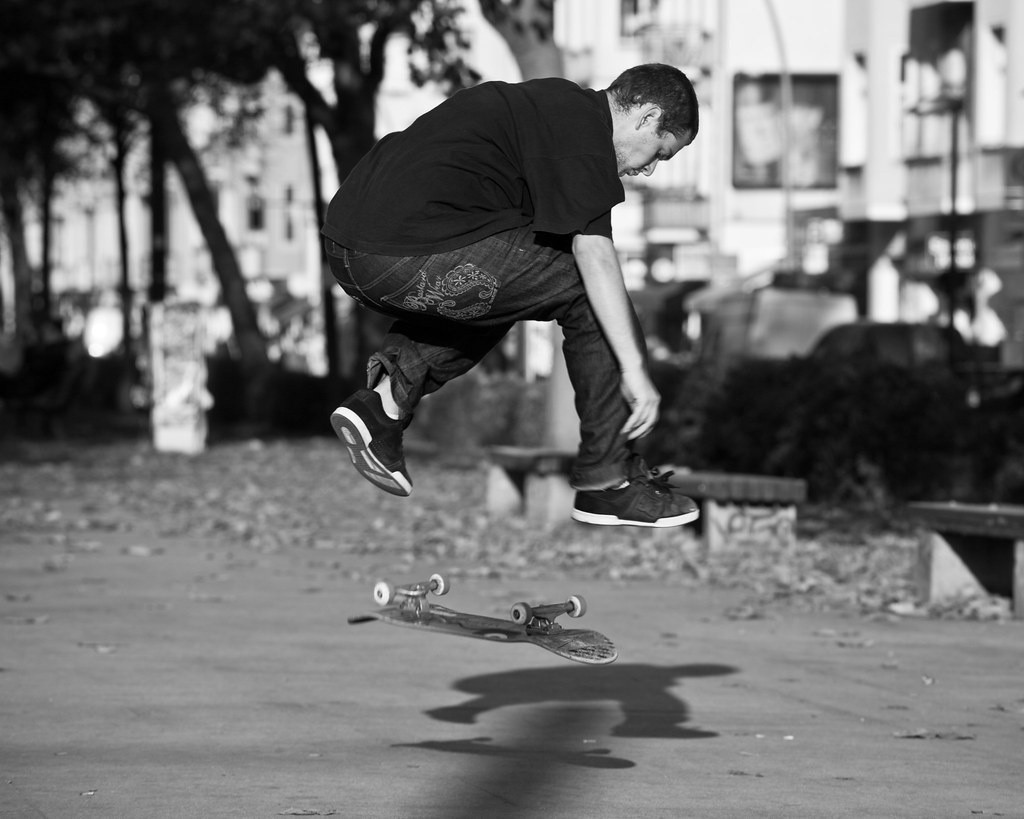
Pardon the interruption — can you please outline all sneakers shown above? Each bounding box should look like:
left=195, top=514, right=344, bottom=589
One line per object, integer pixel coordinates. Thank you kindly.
left=572, top=469, right=699, bottom=527
left=329, top=391, right=414, bottom=496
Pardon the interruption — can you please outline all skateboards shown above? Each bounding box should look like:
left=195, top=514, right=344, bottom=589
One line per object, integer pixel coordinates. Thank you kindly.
left=339, top=570, right=618, bottom=666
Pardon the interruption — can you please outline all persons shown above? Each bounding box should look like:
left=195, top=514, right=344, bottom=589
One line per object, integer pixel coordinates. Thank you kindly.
left=324, top=63, right=699, bottom=528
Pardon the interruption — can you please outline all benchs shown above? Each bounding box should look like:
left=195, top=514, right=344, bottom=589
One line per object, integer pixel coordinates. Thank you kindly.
left=483, top=445, right=579, bottom=521
left=668, top=474, right=805, bottom=550
left=910, top=501, right=1024, bottom=618
